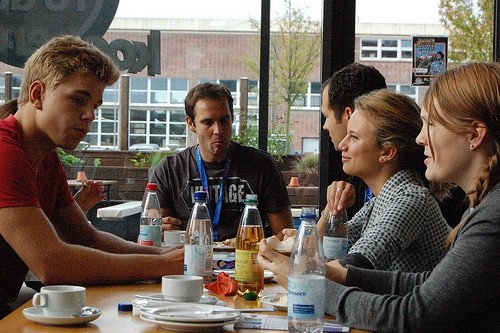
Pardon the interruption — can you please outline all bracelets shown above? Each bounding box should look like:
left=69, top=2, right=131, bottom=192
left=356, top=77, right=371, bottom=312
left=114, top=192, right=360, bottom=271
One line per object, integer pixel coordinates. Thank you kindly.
left=321, top=211, right=328, bottom=219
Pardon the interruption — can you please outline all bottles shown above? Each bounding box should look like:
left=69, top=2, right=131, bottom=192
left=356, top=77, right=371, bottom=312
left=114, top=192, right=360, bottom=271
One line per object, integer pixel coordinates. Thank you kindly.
left=137, top=182, right=163, bottom=248
left=235, top=194, right=265, bottom=297
left=288, top=207, right=326, bottom=333
left=182, top=190, right=214, bottom=284
left=322, top=204, right=348, bottom=263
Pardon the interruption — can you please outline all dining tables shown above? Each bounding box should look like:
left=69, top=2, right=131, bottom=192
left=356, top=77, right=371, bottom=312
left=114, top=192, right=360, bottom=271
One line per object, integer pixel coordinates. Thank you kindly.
left=0, top=278, right=370, bottom=333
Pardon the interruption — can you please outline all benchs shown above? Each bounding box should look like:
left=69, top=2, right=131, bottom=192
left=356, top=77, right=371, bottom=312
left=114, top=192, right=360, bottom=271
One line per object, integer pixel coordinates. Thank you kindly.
left=97, top=200, right=143, bottom=241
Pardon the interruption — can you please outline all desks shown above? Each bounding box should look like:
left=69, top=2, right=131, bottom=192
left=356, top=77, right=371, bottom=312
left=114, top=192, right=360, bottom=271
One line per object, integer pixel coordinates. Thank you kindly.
left=67, top=179, right=117, bottom=235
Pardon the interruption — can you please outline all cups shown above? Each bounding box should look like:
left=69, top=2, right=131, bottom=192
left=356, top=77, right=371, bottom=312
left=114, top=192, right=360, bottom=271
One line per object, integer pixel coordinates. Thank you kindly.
left=160, top=274, right=204, bottom=301
left=31, top=286, right=87, bottom=317
left=163, top=230, right=186, bottom=245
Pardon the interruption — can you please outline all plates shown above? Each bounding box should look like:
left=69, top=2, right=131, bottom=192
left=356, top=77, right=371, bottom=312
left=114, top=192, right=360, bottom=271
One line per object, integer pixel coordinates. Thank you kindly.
left=139, top=303, right=240, bottom=323
left=213, top=269, right=275, bottom=282
left=260, top=292, right=288, bottom=314
left=214, top=242, right=236, bottom=249
left=131, top=294, right=219, bottom=308
left=21, top=306, right=102, bottom=325
left=139, top=315, right=240, bottom=332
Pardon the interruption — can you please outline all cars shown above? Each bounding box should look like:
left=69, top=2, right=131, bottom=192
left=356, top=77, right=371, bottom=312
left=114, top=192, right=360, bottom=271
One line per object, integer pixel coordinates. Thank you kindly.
left=130, top=143, right=159, bottom=150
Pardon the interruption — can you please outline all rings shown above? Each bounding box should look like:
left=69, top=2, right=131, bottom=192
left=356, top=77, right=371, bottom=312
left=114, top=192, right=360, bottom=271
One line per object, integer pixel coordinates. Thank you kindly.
left=337, top=189, right=342, bottom=191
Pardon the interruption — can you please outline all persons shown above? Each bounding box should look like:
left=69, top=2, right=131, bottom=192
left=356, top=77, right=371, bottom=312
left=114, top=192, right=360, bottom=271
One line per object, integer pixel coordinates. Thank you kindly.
left=0, top=99, right=107, bottom=213
left=281, top=62, right=387, bottom=241
left=137, top=82, right=294, bottom=241
left=306, top=88, right=454, bottom=272
left=0, top=35, right=184, bottom=321
left=258, top=61, right=500, bottom=333
